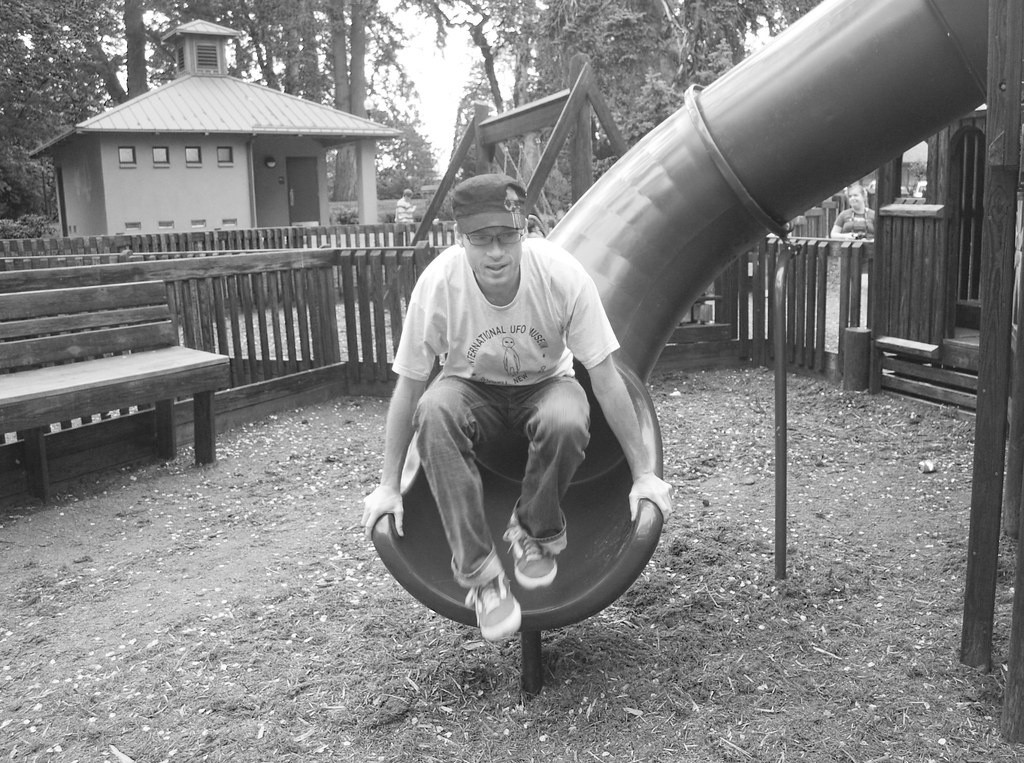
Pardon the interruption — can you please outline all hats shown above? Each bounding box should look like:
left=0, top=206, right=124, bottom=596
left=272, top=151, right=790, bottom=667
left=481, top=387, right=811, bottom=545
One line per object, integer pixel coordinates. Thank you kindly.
left=451, top=173, right=527, bottom=234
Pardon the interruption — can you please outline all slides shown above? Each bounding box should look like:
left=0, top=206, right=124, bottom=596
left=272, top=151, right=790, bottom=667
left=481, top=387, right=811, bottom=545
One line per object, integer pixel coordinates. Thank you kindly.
left=369, top=0, right=989, bottom=632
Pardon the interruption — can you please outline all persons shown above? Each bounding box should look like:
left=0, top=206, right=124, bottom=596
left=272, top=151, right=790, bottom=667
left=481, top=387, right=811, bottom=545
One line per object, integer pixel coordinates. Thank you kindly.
left=831, top=184, right=875, bottom=286
left=360, top=174, right=674, bottom=641
left=395, top=189, right=417, bottom=223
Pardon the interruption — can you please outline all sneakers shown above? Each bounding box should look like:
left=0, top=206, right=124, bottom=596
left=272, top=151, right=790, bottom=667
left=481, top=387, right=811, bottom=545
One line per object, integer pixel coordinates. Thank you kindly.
left=465, top=570, right=521, bottom=640
left=502, top=513, right=558, bottom=590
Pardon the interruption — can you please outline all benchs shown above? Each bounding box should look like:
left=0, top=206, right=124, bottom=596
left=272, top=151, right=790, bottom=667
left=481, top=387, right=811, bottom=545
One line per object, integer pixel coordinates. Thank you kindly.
left=0, top=280, right=229, bottom=503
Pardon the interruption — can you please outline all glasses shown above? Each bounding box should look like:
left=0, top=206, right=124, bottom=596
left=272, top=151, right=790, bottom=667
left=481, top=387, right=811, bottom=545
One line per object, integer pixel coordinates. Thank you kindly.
left=466, top=228, right=525, bottom=246
